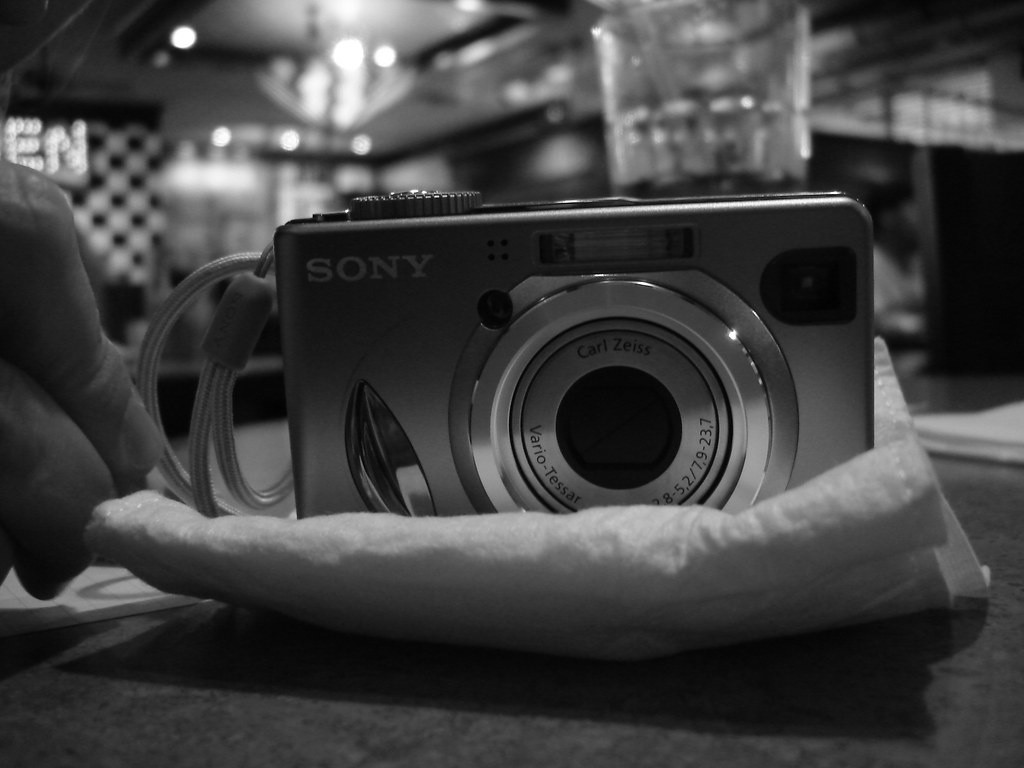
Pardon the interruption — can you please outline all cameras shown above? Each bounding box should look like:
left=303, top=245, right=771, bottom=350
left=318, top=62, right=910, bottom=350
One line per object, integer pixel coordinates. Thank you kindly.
left=273, top=186, right=876, bottom=525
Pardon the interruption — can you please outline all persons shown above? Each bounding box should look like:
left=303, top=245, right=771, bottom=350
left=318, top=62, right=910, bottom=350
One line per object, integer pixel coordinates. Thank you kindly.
left=0, top=155, right=166, bottom=600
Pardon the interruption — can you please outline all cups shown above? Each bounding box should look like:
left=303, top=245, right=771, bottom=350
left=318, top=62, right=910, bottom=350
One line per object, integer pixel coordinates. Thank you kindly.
left=592, top=0, right=811, bottom=197
